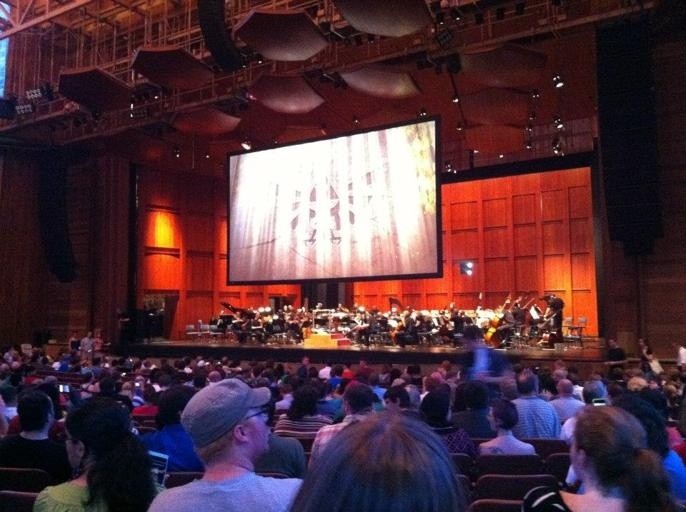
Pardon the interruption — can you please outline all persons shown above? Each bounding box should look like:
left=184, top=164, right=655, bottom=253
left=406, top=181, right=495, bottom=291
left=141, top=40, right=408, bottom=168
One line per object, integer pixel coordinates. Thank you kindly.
left=0, top=336, right=686, bottom=512
left=209, top=295, right=565, bottom=350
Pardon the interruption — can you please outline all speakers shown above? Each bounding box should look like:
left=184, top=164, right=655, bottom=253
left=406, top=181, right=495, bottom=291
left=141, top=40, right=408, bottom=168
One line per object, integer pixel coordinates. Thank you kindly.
left=595, top=22, right=665, bottom=253
left=39, top=167, right=78, bottom=282
left=198, top=0, right=243, bottom=73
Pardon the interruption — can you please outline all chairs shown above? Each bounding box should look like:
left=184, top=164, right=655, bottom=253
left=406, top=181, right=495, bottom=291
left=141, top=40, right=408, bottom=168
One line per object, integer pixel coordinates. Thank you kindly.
left=0, top=354, right=686, bottom=511
left=183, top=312, right=589, bottom=349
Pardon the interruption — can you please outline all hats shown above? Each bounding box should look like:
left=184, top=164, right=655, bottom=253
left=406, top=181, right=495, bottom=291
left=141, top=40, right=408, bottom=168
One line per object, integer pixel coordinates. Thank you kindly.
left=179, top=378, right=273, bottom=449
